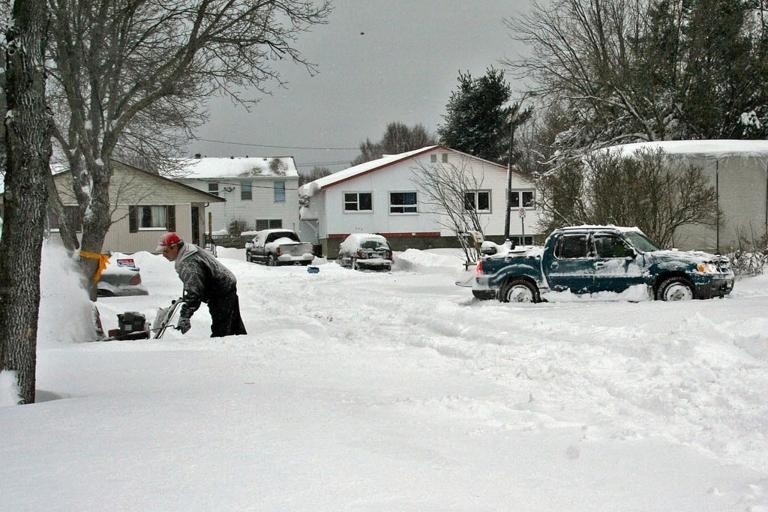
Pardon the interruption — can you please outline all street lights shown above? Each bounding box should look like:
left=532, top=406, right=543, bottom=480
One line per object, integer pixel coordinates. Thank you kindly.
left=503, top=89, right=537, bottom=243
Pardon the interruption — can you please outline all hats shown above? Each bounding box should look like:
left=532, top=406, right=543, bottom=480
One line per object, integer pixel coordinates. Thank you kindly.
left=150, top=232, right=182, bottom=254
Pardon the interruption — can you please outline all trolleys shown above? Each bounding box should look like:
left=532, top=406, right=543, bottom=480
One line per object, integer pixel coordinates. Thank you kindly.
left=88, top=301, right=185, bottom=340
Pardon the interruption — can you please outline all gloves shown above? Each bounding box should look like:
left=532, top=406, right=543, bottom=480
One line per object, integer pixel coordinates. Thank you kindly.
left=177, top=317, right=191, bottom=334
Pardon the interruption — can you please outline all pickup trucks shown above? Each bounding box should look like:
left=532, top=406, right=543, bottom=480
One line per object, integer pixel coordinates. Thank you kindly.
left=245, top=228, right=315, bottom=267
left=472, top=223, right=737, bottom=303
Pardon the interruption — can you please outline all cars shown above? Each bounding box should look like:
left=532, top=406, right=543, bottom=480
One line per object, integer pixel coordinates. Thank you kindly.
left=337, top=231, right=396, bottom=274
left=92, top=263, right=149, bottom=296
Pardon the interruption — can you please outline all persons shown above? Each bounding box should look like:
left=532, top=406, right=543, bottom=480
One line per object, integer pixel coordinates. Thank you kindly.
left=154, top=232, right=248, bottom=336
